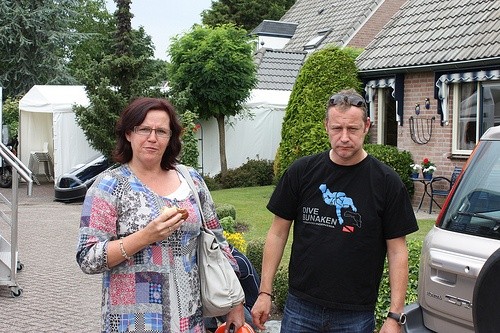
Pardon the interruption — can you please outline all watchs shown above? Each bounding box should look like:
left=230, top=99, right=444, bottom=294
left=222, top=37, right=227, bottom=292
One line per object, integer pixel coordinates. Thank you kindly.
left=387, top=312, right=408, bottom=325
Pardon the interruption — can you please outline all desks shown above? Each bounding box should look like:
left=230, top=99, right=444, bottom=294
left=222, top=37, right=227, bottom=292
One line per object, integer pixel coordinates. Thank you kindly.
left=410, top=177, right=443, bottom=215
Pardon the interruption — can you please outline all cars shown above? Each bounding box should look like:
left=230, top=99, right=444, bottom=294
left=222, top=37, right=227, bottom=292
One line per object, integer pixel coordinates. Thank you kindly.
left=55, top=151, right=109, bottom=202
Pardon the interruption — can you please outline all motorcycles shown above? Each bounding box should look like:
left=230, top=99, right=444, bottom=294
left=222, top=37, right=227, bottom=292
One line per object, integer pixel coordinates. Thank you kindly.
left=0, top=144, right=16, bottom=186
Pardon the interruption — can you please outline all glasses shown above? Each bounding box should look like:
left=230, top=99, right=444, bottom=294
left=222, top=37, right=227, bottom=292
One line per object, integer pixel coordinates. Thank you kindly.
left=130, top=125, right=172, bottom=138
left=329, top=95, right=368, bottom=110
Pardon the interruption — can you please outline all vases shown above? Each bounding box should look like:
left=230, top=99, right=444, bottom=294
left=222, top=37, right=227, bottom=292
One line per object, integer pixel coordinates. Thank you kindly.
left=423, top=171, right=432, bottom=179
left=410, top=172, right=420, bottom=179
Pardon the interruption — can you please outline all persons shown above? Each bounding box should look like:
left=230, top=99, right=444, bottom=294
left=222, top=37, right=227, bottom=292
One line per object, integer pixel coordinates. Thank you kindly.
left=76, top=97, right=246, bottom=333
left=250, top=88, right=419, bottom=333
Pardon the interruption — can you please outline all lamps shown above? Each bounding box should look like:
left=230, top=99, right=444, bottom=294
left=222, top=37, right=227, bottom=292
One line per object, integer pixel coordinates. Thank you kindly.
left=414, top=102, right=421, bottom=115
left=424, top=98, right=430, bottom=110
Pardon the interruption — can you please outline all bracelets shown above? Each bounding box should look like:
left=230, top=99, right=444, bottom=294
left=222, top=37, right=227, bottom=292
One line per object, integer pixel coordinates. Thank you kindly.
left=120, top=237, right=132, bottom=260
left=258, top=291, right=275, bottom=301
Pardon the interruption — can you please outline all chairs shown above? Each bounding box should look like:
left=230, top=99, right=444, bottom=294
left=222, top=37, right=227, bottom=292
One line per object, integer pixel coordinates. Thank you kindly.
left=424, top=166, right=463, bottom=215
left=28, top=141, right=54, bottom=185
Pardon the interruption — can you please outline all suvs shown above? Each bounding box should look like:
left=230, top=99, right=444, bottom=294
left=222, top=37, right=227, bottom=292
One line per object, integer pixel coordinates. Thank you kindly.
left=417, top=125, right=500, bottom=333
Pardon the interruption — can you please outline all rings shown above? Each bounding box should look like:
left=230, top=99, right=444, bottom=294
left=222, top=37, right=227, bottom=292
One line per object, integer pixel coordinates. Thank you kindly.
left=169, top=227, right=172, bottom=232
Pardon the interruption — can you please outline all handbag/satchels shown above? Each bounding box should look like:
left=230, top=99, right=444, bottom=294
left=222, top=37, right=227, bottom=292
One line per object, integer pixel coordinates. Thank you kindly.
left=177, top=165, right=245, bottom=317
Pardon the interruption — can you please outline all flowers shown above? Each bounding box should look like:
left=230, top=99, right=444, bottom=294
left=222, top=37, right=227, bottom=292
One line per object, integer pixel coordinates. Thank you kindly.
left=421, top=158, right=438, bottom=174
left=409, top=163, right=423, bottom=174
